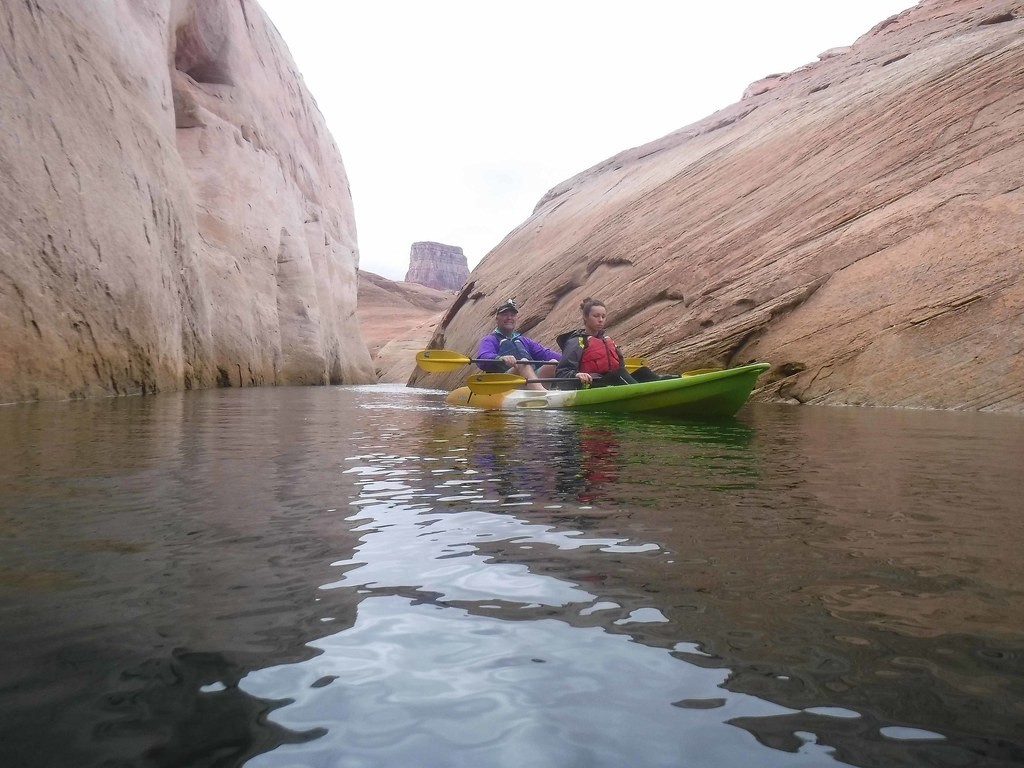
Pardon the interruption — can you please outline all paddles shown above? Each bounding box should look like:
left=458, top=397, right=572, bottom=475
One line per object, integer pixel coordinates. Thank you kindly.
left=414, top=349, right=647, bottom=374
left=465, top=367, right=722, bottom=396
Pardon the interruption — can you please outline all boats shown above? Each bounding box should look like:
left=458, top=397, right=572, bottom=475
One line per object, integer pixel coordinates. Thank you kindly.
left=443, top=360, right=771, bottom=421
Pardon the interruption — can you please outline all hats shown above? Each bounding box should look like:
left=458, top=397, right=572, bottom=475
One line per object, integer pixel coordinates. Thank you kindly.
left=496, top=304, right=518, bottom=315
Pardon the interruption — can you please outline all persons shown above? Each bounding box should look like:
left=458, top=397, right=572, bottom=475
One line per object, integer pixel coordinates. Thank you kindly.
left=555, top=297, right=681, bottom=390
left=475, top=302, right=562, bottom=390
